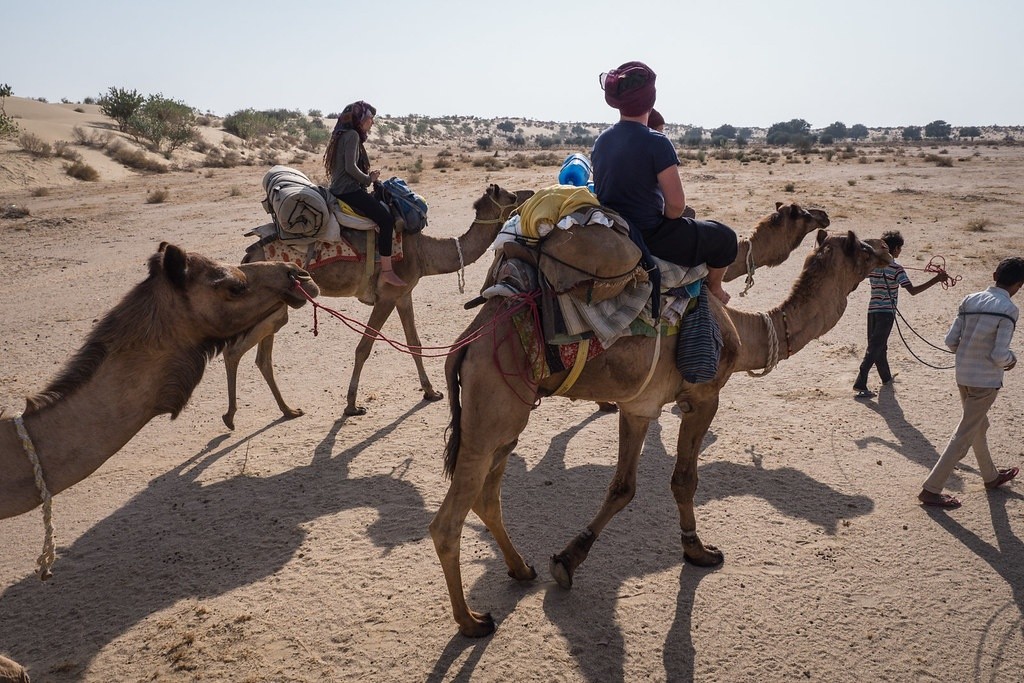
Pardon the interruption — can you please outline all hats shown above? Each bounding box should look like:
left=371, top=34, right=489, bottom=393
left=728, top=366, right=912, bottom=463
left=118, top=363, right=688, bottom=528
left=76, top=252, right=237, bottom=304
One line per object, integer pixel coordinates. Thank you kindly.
left=648, top=109, right=665, bottom=128
left=599, top=61, right=656, bottom=117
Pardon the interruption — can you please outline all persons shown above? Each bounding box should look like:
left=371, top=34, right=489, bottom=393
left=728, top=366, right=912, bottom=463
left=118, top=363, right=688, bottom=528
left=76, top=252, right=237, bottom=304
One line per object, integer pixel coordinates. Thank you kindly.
left=647, top=108, right=696, bottom=219
left=591, top=61, right=739, bottom=307
left=323, top=101, right=408, bottom=288
left=853, top=230, right=948, bottom=397
left=916, top=258, right=1024, bottom=510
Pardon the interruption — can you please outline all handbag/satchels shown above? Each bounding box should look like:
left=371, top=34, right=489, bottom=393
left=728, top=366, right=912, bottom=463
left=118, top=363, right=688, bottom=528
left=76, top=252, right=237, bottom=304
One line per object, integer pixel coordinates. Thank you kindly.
left=676, top=307, right=724, bottom=384
left=537, top=226, right=642, bottom=303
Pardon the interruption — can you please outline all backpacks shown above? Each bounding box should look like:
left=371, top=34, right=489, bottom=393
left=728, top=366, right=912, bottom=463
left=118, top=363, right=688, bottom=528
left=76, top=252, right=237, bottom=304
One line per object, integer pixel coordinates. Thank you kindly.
left=374, top=177, right=428, bottom=234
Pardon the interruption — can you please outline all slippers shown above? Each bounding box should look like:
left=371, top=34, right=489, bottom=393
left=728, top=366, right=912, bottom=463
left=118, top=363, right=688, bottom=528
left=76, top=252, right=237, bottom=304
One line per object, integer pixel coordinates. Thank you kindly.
left=993, top=467, right=1019, bottom=489
left=924, top=494, right=961, bottom=508
left=853, top=391, right=877, bottom=397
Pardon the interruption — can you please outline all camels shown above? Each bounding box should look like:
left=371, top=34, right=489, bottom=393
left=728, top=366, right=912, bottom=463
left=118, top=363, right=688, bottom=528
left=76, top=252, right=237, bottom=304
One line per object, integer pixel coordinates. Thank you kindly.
left=222, top=183, right=536, bottom=431
left=426, top=230, right=892, bottom=640
left=0, top=240, right=320, bottom=523
left=595, top=201, right=829, bottom=415
